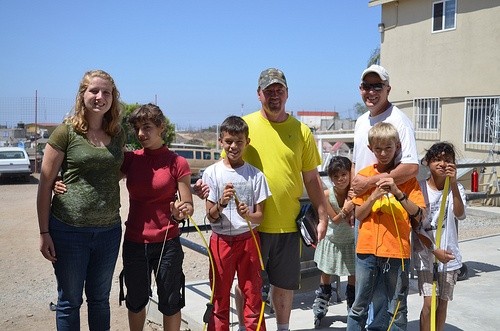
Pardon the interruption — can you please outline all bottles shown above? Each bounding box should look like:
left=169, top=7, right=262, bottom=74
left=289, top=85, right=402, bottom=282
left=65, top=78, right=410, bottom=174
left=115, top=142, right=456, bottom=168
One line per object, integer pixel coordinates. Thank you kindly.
left=471, top=167, right=479, bottom=192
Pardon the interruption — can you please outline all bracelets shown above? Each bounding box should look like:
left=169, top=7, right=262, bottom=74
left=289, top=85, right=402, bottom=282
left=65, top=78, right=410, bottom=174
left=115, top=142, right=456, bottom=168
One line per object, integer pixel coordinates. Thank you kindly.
left=38, top=231, right=50, bottom=236
left=170, top=211, right=185, bottom=225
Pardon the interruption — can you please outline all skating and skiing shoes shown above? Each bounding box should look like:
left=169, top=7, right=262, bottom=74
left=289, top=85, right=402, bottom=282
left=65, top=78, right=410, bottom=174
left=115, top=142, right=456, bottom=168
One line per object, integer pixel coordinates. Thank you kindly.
left=345, top=285, right=356, bottom=313
left=313, top=285, right=332, bottom=328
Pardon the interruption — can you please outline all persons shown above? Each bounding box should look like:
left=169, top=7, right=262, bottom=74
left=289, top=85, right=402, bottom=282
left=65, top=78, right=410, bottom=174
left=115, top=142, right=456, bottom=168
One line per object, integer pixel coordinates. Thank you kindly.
left=414, top=141, right=468, bottom=331
left=313, top=155, right=360, bottom=318
left=201, top=112, right=273, bottom=331
left=346, top=121, right=427, bottom=331
left=35, top=70, right=134, bottom=331
left=52, top=103, right=196, bottom=331
left=192, top=66, right=329, bottom=330
left=348, top=63, right=422, bottom=331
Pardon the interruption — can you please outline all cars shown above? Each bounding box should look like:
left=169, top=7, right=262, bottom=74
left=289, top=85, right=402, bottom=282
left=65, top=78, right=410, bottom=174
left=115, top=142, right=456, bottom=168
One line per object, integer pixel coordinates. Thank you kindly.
left=1, top=129, right=52, bottom=150
left=0, top=146, right=31, bottom=182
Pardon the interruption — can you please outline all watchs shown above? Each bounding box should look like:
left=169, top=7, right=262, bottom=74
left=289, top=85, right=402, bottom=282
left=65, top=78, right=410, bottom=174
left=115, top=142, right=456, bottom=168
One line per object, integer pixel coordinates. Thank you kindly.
left=395, top=192, right=406, bottom=202
left=217, top=196, right=228, bottom=208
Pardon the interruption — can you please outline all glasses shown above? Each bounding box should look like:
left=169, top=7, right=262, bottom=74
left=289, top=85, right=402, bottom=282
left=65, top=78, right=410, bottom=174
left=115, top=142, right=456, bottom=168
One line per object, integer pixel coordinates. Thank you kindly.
left=362, top=82, right=387, bottom=90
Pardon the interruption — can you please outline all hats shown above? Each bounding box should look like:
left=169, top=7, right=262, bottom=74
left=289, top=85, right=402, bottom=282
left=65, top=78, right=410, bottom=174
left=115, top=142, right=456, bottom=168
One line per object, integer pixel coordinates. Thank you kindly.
left=361, top=65, right=390, bottom=83
left=259, top=68, right=287, bottom=90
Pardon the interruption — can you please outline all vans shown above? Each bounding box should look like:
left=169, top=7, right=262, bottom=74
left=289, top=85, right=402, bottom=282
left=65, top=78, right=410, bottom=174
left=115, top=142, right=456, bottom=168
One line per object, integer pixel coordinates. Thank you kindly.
left=168, top=147, right=223, bottom=181
